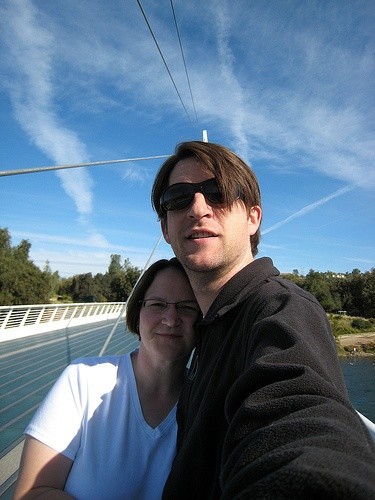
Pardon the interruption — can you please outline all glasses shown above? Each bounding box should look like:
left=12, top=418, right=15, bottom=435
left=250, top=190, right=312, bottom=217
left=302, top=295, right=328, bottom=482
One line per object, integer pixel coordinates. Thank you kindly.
left=159, top=177, right=246, bottom=216
left=139, top=299, right=199, bottom=317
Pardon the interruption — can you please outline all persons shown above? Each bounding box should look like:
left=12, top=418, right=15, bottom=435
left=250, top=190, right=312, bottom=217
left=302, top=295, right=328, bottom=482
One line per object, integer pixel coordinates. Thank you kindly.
left=7, top=258, right=211, bottom=500
left=149, top=138, right=375, bottom=499
